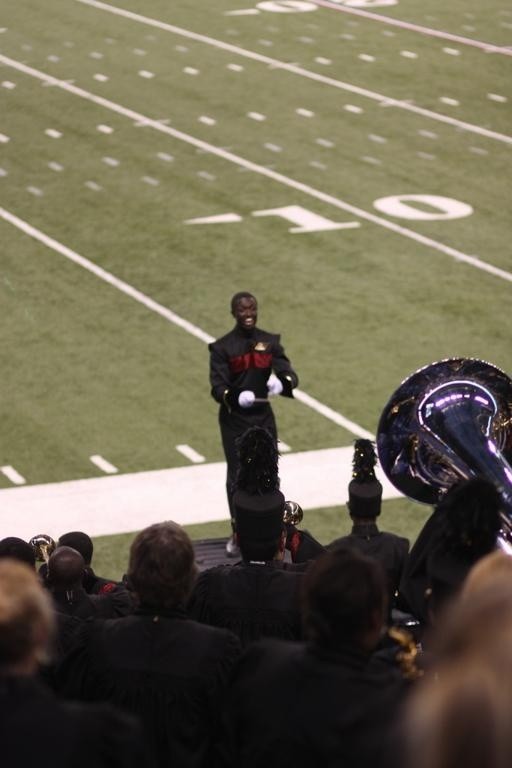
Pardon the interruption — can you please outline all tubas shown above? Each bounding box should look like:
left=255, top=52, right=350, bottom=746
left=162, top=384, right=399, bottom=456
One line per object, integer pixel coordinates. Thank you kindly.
left=376, top=357, right=512, bottom=555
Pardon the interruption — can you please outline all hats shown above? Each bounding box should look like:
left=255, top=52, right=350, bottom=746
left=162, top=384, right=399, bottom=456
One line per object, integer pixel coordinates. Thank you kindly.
left=233, top=488, right=285, bottom=537
left=347, top=477, right=382, bottom=517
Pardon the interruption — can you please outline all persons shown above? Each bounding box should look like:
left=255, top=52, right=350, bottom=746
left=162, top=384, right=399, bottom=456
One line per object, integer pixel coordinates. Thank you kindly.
left=396, top=479, right=502, bottom=642
left=0, top=476, right=418, bottom=768
left=207, top=291, right=300, bottom=559
left=391, top=544, right=512, bottom=768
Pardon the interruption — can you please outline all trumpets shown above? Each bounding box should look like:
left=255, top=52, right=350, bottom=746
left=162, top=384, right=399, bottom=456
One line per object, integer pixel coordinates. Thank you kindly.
left=284, top=501, right=303, bottom=526
left=30, top=535, right=55, bottom=568
